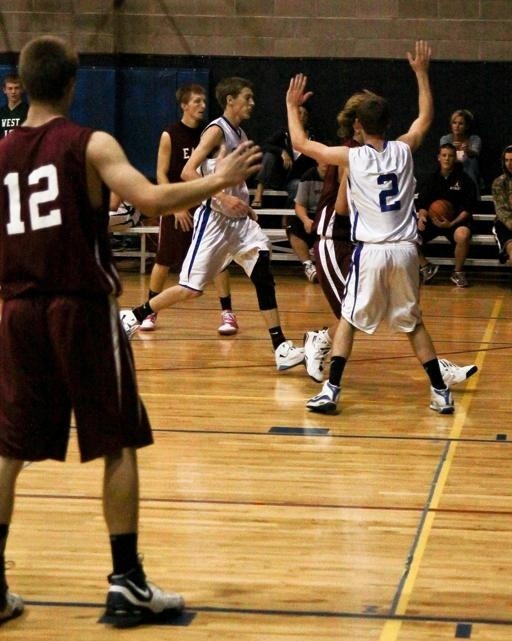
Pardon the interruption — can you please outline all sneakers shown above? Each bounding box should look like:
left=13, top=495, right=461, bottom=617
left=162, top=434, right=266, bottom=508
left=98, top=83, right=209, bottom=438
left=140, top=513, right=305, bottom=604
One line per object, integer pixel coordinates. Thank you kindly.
left=106, top=564, right=188, bottom=627
left=438, top=358, right=477, bottom=387
left=140, top=312, right=157, bottom=331
left=304, top=262, right=317, bottom=283
left=0, top=593, right=23, bottom=621
left=218, top=310, right=239, bottom=336
left=274, top=339, right=306, bottom=371
left=422, top=264, right=439, bottom=285
left=306, top=381, right=341, bottom=413
left=303, top=327, right=331, bottom=383
left=429, top=384, right=454, bottom=414
left=120, top=310, right=139, bottom=342
left=450, top=272, right=469, bottom=289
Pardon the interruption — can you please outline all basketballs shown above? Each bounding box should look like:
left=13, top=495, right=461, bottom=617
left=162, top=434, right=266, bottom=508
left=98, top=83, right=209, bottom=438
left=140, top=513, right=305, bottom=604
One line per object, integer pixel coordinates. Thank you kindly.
left=429, top=199, right=453, bottom=222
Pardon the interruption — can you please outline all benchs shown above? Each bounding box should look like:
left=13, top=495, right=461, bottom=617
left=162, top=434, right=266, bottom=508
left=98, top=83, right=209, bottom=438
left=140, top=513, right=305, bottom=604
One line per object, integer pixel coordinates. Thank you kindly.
left=107, top=179, right=315, bottom=274
left=415, top=194, right=511, bottom=267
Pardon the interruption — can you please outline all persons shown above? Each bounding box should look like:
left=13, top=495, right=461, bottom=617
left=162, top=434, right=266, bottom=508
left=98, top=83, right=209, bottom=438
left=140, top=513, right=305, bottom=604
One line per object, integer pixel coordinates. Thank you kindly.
left=2, top=76, right=26, bottom=139
left=284, top=39, right=458, bottom=414
left=119, top=77, right=305, bottom=372
left=1, top=37, right=264, bottom=628
left=138, top=84, right=239, bottom=334
left=284, top=156, right=330, bottom=283
left=491, top=145, right=512, bottom=267
left=251, top=103, right=316, bottom=210
left=440, top=109, right=481, bottom=200
left=303, top=90, right=477, bottom=386
left=416, top=143, right=477, bottom=288
left=107, top=188, right=142, bottom=232
